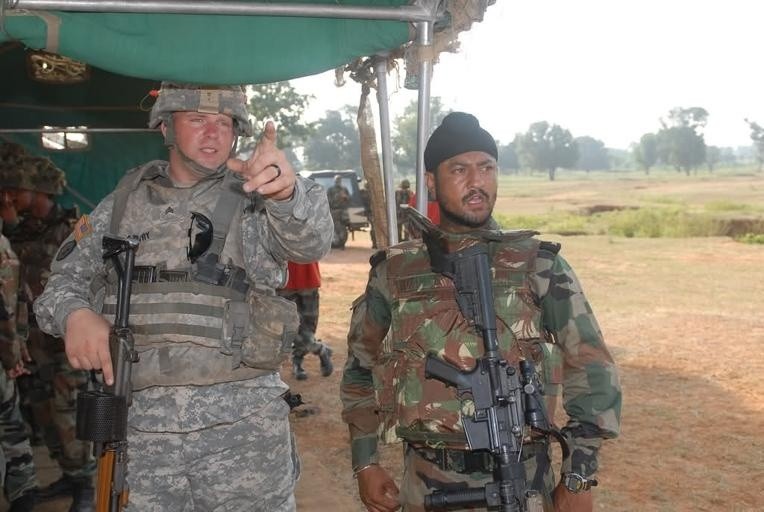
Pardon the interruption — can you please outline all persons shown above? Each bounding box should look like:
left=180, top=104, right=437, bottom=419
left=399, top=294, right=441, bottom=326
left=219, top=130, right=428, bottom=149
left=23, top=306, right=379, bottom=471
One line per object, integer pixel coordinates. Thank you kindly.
left=361, top=174, right=376, bottom=249
left=279, top=262, right=334, bottom=383
left=340, top=111, right=623, bottom=512
left=33, top=79, right=334, bottom=512
left=2, top=131, right=98, bottom=512
left=396, top=178, right=414, bottom=240
left=328, top=174, right=349, bottom=249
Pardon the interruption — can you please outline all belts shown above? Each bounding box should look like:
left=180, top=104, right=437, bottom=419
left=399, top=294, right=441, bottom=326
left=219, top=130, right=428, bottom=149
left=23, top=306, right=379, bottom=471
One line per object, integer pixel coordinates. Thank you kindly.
left=415, top=445, right=534, bottom=472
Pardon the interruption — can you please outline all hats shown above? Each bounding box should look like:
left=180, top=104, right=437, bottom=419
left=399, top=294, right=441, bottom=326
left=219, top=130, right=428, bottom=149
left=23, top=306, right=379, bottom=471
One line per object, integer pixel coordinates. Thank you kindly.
left=424, top=112, right=498, bottom=170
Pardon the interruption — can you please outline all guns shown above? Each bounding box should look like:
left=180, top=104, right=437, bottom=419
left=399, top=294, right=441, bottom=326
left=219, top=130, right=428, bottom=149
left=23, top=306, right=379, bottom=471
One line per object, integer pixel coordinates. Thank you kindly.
left=424, top=242, right=549, bottom=512
left=76, top=233, right=140, bottom=512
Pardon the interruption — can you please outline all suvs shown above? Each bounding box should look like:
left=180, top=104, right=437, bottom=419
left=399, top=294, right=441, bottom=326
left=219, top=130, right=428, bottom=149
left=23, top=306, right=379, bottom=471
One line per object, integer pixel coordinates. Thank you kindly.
left=297, top=168, right=369, bottom=249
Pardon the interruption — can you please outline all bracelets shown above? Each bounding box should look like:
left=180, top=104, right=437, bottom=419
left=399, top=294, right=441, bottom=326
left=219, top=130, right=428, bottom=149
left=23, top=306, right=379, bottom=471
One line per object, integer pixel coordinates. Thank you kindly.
left=353, top=464, right=374, bottom=480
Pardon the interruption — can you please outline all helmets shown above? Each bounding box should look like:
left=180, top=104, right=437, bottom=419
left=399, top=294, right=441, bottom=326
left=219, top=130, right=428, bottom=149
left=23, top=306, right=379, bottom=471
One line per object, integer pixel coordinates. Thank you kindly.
left=148, top=83, right=252, bottom=137
left=5, top=155, right=64, bottom=195
left=0, top=143, right=26, bottom=167
left=399, top=180, right=410, bottom=188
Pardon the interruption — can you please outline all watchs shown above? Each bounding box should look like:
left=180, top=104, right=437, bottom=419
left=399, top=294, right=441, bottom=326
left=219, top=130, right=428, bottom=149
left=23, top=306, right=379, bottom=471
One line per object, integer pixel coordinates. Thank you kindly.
left=561, top=472, right=598, bottom=495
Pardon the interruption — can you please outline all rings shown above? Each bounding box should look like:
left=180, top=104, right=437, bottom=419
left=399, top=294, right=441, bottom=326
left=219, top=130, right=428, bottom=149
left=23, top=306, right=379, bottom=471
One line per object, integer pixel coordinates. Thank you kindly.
left=270, top=163, right=280, bottom=177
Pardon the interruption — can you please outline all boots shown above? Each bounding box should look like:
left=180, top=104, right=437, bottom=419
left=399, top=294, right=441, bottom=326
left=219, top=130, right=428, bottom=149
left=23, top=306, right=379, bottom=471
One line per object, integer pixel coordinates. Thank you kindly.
left=319, top=346, right=333, bottom=377
left=70, top=482, right=96, bottom=512
left=292, top=364, right=306, bottom=379
left=36, top=478, right=71, bottom=499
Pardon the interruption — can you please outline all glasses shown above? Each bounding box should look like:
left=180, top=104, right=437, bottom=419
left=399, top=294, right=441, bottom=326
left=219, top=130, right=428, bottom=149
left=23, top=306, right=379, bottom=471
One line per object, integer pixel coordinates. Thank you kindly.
left=188, top=211, right=213, bottom=263
